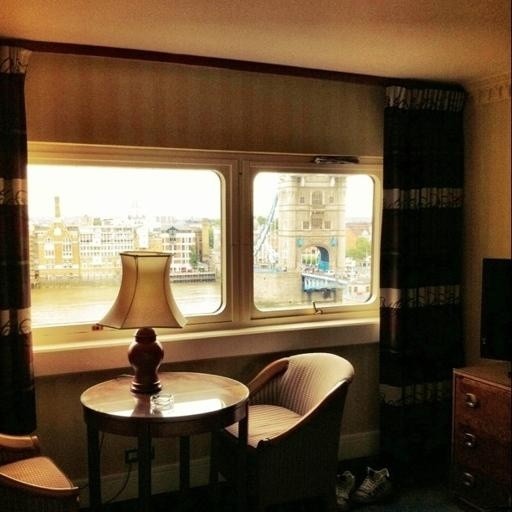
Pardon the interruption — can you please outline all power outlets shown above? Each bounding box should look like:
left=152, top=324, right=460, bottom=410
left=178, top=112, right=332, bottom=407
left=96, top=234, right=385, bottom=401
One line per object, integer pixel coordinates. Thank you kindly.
left=125, top=447, right=155, bottom=462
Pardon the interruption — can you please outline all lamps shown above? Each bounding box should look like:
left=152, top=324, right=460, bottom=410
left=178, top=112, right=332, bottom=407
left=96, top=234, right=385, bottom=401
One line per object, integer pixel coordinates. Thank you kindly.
left=97, top=251, right=187, bottom=393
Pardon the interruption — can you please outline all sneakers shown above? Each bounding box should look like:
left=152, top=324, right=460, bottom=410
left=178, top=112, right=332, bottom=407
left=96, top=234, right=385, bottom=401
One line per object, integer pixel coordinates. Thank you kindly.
left=336, top=471, right=356, bottom=505
left=356, top=465, right=389, bottom=498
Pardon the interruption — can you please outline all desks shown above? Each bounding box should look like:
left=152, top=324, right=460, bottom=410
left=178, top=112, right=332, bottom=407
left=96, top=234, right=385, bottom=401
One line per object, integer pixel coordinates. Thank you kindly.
left=80, top=371, right=249, bottom=512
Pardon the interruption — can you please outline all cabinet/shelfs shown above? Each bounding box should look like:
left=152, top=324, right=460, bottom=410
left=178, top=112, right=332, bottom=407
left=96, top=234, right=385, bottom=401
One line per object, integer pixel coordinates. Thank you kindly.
left=451, top=358, right=512, bottom=509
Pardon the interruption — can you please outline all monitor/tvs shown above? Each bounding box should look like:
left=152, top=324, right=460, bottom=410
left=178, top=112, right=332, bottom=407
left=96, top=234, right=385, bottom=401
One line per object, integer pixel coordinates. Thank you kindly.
left=480, top=259, right=512, bottom=377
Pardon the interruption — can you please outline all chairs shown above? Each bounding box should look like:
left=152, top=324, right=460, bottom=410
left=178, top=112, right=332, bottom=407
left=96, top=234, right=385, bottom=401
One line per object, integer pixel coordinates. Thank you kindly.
left=209, top=352, right=355, bottom=511
left=1, top=434, right=80, bottom=512
